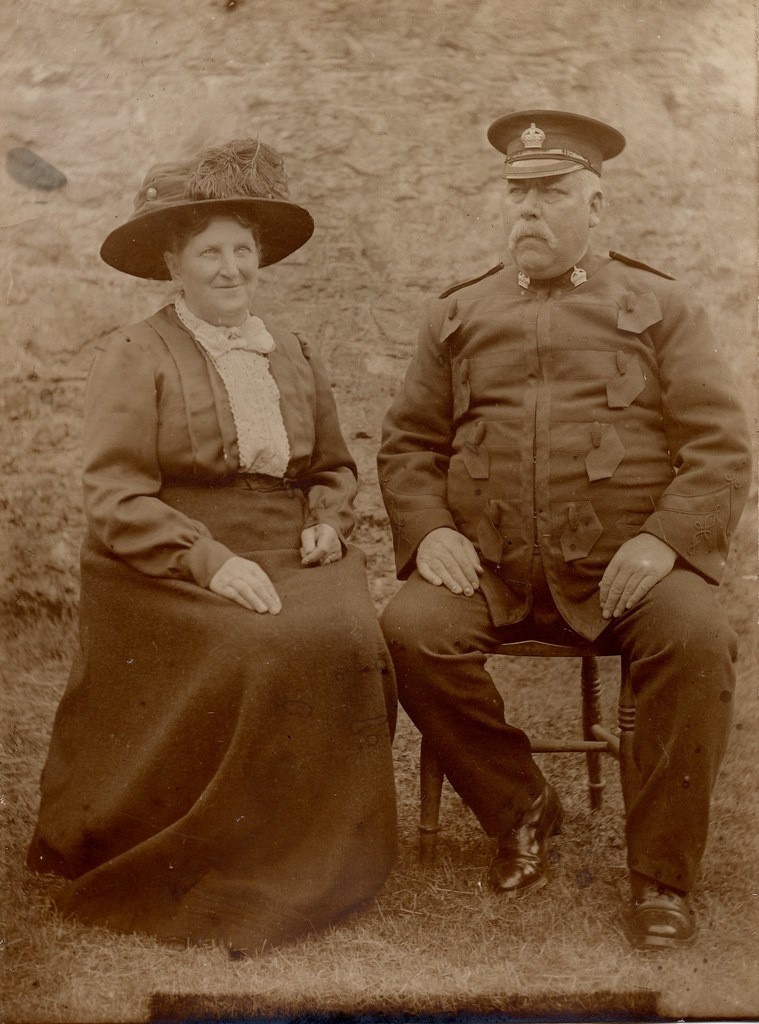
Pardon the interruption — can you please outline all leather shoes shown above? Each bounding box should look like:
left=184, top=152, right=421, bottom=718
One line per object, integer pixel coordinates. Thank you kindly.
left=485, top=781, right=567, bottom=901
left=627, top=872, right=699, bottom=949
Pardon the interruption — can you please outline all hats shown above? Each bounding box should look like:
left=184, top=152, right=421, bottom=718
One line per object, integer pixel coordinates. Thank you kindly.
left=485, top=110, right=627, bottom=180
left=99, top=137, right=315, bottom=281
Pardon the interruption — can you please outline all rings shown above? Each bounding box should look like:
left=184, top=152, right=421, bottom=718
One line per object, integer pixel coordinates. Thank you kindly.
left=328, top=556, right=332, bottom=563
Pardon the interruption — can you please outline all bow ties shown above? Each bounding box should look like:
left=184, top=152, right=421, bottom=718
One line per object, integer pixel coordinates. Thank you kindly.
left=193, top=316, right=277, bottom=360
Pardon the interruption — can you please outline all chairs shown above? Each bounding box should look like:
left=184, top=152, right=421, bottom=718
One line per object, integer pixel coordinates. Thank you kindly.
left=416, top=639, right=637, bottom=874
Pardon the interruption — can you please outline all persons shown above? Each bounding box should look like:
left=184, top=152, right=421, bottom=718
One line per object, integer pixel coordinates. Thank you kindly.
left=376, top=109, right=753, bottom=944
left=31, top=139, right=399, bottom=953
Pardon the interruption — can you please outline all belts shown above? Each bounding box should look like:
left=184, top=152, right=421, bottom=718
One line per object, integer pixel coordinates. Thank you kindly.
left=201, top=473, right=302, bottom=494
